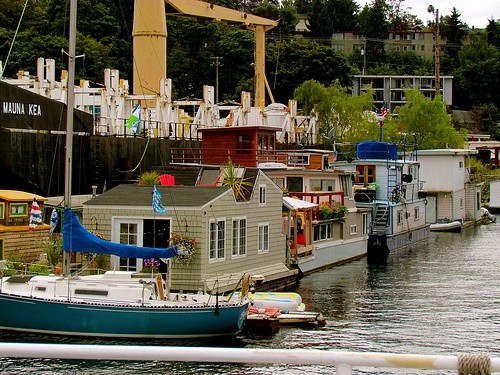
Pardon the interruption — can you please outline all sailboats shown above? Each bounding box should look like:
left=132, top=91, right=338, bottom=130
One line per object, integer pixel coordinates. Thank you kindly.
left=0, top=0, right=252, bottom=338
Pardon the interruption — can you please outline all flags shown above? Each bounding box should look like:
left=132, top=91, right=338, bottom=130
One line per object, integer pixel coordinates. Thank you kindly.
left=28, top=194, right=42, bottom=233
left=49, top=209, right=59, bottom=236
left=153, top=185, right=167, bottom=213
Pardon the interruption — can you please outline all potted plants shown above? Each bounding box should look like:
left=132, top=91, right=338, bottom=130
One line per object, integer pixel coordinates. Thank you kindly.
left=319, top=201, right=348, bottom=221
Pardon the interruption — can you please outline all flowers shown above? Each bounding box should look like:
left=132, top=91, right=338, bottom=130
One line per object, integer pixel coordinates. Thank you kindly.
left=167, top=234, right=195, bottom=265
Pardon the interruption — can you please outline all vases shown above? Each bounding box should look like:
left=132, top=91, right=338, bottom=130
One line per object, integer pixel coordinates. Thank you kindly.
left=177, top=250, right=188, bottom=255
